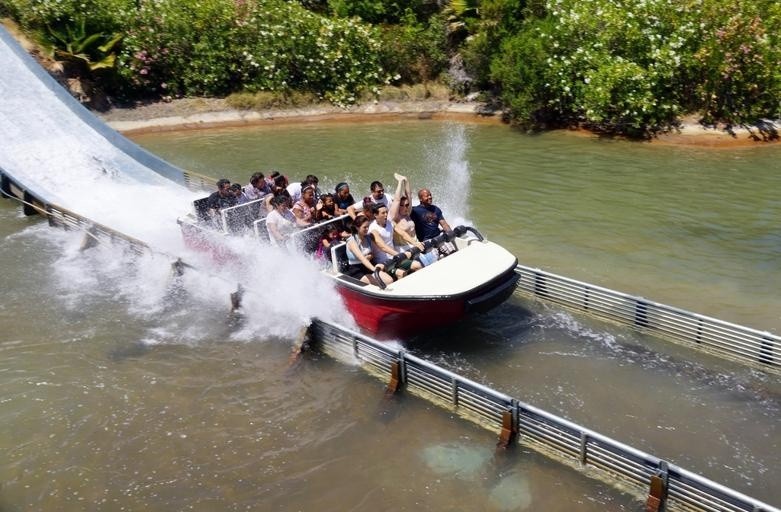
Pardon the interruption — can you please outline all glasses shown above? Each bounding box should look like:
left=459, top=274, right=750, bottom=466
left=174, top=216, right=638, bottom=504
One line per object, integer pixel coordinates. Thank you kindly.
left=400, top=203, right=410, bottom=207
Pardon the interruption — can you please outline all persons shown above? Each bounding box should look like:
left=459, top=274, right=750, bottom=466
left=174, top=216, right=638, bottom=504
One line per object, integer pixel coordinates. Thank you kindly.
left=204, top=170, right=452, bottom=284
